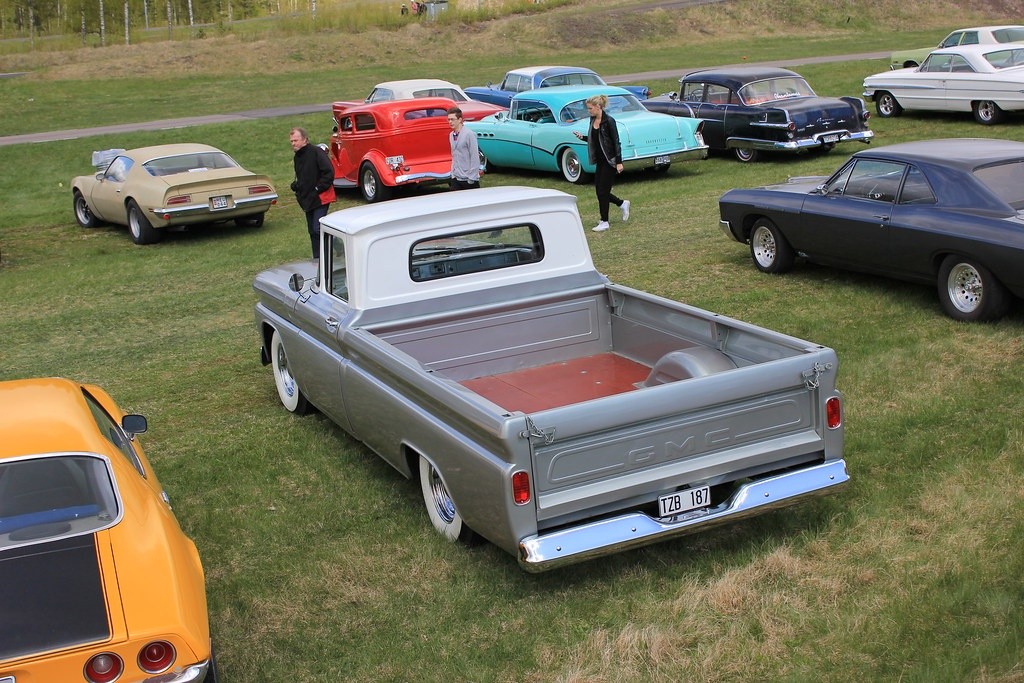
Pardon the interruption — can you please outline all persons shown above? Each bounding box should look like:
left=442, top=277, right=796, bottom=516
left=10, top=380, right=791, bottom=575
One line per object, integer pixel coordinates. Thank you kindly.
left=448, top=107, right=480, bottom=190
left=289, top=127, right=336, bottom=259
left=411, top=0, right=427, bottom=18
left=576, top=94, right=630, bottom=231
left=401, top=4, right=408, bottom=16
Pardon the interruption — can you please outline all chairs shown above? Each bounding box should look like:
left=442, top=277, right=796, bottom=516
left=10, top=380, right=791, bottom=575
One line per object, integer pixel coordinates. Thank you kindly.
left=10, top=485, right=80, bottom=516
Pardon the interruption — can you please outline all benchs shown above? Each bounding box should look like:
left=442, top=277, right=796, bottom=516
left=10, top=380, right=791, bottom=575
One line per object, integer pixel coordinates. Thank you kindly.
left=412, top=243, right=533, bottom=277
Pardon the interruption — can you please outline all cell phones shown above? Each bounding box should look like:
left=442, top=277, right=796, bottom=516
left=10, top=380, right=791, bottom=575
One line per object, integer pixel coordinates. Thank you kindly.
left=573, top=131, right=581, bottom=137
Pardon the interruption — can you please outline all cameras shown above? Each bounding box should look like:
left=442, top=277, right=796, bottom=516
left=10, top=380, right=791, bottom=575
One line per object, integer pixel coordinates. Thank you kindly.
left=291, top=182, right=300, bottom=191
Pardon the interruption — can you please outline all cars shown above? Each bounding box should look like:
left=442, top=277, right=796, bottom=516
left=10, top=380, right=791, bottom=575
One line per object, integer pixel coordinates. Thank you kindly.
left=289, top=97, right=463, bottom=204
left=464, top=83, right=710, bottom=185
left=621, top=66, right=875, bottom=164
left=1, top=376, right=220, bottom=683
left=719, top=136, right=1024, bottom=322
left=462, top=64, right=653, bottom=115
left=889, top=24, right=1024, bottom=70
left=362, top=78, right=509, bottom=125
left=862, top=43, right=1024, bottom=126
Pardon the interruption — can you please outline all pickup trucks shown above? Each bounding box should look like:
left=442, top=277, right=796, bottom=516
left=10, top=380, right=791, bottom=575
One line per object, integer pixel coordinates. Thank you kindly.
left=251, top=182, right=852, bottom=576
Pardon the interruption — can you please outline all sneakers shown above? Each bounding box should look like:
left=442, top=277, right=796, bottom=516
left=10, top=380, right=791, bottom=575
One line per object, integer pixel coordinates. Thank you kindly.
left=592, top=219, right=609, bottom=232
left=620, top=200, right=630, bottom=221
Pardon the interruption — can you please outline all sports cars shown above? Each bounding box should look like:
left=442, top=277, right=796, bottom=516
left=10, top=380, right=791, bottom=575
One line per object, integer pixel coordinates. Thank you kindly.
left=69, top=142, right=280, bottom=245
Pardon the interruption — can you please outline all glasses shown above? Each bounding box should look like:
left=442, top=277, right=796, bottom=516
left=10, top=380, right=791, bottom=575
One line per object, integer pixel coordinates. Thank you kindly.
left=447, top=118, right=460, bottom=122
